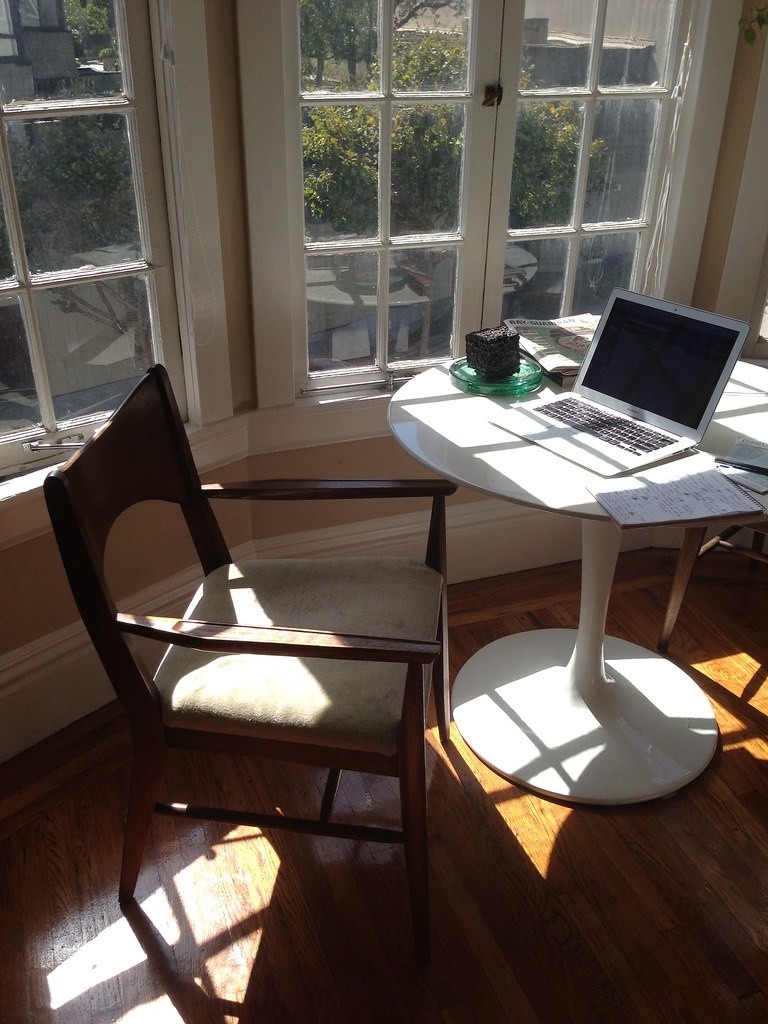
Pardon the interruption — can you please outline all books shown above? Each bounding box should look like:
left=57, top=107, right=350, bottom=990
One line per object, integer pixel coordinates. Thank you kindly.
left=587, top=463, right=767, bottom=529
left=504, top=311, right=602, bottom=386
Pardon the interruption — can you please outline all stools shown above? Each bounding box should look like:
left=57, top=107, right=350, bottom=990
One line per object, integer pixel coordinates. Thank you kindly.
left=658, top=520, right=768, bottom=652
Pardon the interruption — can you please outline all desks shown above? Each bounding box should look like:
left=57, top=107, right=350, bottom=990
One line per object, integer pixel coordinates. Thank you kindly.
left=301, top=218, right=537, bottom=362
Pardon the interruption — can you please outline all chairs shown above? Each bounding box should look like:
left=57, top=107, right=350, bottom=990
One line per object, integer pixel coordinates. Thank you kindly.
left=388, top=339, right=768, bottom=807
left=42, top=363, right=459, bottom=950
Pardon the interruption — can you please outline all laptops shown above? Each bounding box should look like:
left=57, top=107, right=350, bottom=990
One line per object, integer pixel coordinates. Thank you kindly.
left=489, top=287, right=752, bottom=477
